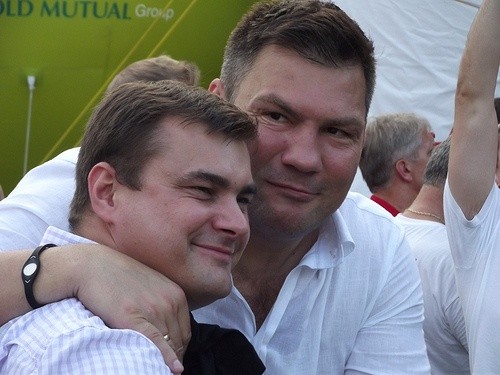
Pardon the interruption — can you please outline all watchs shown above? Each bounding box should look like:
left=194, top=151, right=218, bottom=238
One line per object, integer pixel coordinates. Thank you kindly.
left=20, top=243, right=58, bottom=310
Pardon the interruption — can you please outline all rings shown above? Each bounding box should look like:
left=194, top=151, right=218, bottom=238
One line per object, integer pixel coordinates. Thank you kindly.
left=163, top=334, right=171, bottom=341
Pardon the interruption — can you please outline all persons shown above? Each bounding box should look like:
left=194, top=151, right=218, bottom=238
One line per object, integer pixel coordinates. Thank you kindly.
left=0, top=54, right=259, bottom=375
left=0, top=0, right=432, bottom=375
left=441, top=0, right=500, bottom=375
left=358, top=96, right=500, bottom=375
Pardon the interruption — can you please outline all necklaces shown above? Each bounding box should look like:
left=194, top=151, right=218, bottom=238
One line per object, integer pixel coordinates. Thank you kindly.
left=405, top=209, right=445, bottom=224
